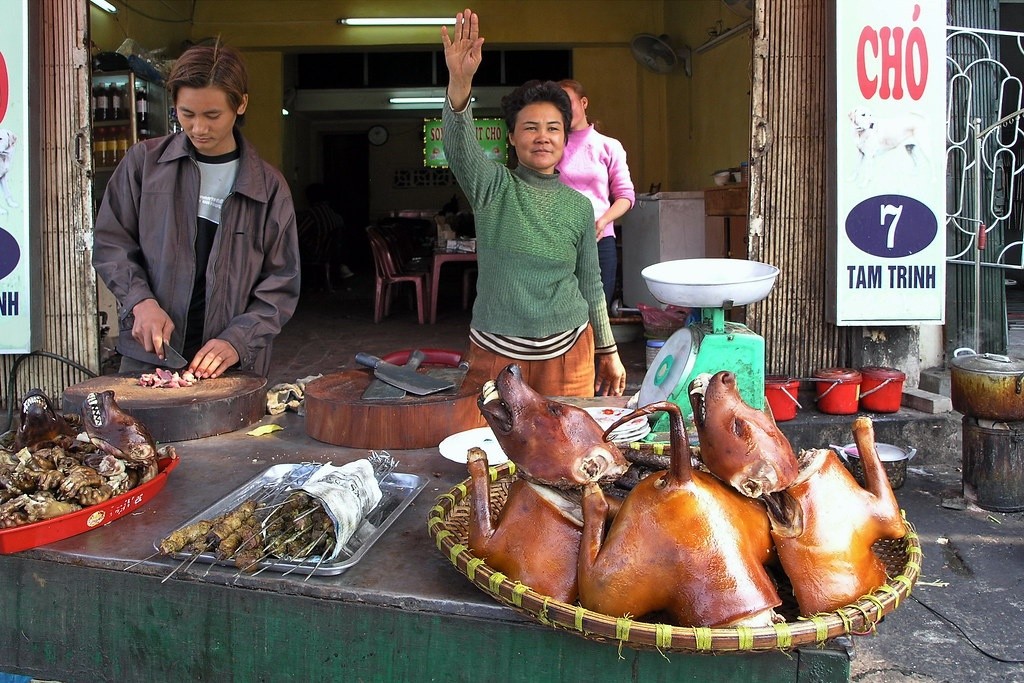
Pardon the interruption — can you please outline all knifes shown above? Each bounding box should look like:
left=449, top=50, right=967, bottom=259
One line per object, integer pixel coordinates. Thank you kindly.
left=355, top=351, right=456, bottom=396
left=162, top=341, right=188, bottom=369
left=360, top=349, right=426, bottom=401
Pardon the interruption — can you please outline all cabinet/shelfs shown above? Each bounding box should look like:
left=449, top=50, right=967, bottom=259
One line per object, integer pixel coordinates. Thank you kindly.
left=91, top=70, right=184, bottom=217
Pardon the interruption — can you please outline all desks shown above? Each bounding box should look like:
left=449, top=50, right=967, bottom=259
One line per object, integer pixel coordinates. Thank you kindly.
left=427, top=243, right=476, bottom=324
left=705, top=184, right=748, bottom=259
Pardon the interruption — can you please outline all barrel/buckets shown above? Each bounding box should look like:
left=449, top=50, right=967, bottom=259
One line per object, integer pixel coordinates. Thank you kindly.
left=858, top=366, right=905, bottom=413
left=812, top=367, right=863, bottom=415
left=765, top=374, right=803, bottom=422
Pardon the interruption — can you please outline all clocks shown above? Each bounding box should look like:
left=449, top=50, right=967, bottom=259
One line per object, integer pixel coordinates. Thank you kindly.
left=368, top=125, right=389, bottom=147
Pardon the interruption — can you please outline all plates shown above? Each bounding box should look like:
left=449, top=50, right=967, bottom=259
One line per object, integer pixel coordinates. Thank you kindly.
left=581, top=406, right=652, bottom=445
left=438, top=425, right=509, bottom=466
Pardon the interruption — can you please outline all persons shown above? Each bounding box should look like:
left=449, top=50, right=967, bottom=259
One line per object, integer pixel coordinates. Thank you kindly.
left=92, top=45, right=303, bottom=379
left=439, top=8, right=630, bottom=401
left=552, top=79, right=637, bottom=310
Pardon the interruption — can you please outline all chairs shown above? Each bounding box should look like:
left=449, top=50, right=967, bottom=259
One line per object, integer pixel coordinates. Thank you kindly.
left=368, top=228, right=430, bottom=324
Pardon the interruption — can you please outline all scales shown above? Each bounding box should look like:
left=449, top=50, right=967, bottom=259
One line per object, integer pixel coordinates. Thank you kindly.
left=636, top=257, right=780, bottom=441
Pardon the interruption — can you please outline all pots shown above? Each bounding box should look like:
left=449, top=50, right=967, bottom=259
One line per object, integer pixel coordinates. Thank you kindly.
left=829, top=442, right=917, bottom=489
left=949, top=347, right=1024, bottom=421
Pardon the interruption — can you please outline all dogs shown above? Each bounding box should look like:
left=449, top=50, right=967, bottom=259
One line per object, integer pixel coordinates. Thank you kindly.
left=467, top=364, right=905, bottom=625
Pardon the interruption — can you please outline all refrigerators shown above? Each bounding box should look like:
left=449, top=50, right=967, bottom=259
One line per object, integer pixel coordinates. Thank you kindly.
left=621, top=192, right=705, bottom=308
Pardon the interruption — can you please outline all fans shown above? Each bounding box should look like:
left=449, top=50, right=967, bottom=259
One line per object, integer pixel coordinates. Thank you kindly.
left=631, top=33, right=692, bottom=78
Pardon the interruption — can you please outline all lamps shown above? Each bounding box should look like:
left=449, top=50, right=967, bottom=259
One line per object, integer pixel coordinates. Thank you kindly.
left=337, top=18, right=465, bottom=26
left=694, top=18, right=752, bottom=55
left=389, top=97, right=476, bottom=104
left=90, top=0, right=118, bottom=15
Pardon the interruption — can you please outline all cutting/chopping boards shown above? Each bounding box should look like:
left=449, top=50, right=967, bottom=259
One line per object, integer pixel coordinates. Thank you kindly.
left=62, top=368, right=269, bottom=443
left=303, top=366, right=491, bottom=451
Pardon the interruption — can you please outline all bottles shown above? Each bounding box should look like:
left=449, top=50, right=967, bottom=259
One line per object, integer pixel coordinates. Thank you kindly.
left=741, top=161, right=747, bottom=183
left=92, top=82, right=150, bottom=167
left=645, top=339, right=665, bottom=371
